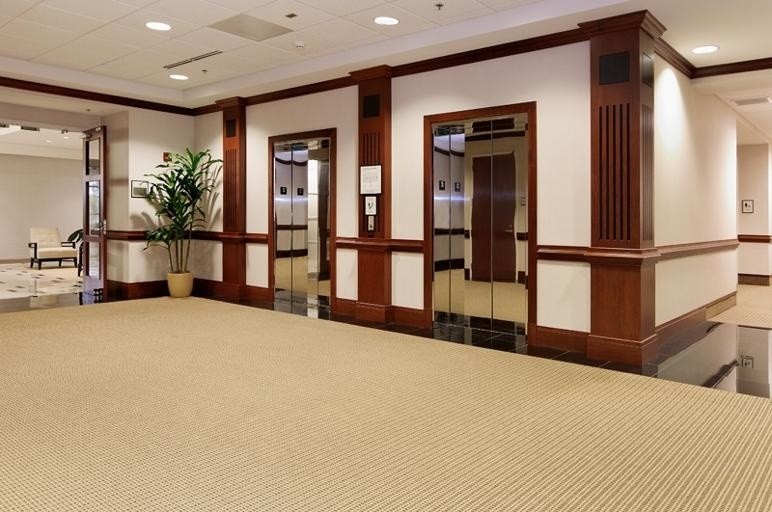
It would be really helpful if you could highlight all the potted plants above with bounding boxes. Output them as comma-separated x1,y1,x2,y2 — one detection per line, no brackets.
139,144,224,298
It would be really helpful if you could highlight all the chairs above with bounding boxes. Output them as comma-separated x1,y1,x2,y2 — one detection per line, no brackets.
28,227,84,276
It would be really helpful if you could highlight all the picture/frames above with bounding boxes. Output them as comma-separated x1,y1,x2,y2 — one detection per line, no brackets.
742,356,753,368
742,200,754,213
